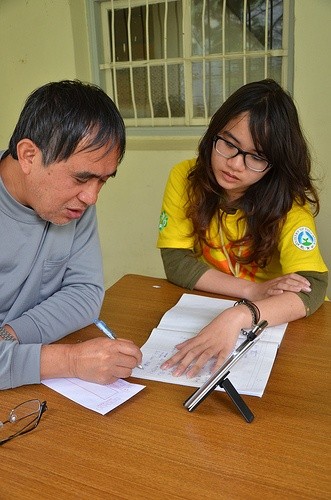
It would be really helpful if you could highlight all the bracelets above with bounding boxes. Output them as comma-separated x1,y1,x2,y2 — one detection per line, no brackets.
0,327,16,342
235,299,260,334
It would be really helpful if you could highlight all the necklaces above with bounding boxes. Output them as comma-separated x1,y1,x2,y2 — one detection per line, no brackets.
217,200,249,279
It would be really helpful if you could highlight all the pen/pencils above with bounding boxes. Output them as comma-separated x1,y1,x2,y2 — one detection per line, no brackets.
93,318,145,372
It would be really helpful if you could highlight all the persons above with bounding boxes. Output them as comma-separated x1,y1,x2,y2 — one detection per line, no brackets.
0,80,142,391
156,80,328,378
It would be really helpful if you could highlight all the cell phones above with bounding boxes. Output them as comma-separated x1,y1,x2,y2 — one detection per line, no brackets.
183,336,260,407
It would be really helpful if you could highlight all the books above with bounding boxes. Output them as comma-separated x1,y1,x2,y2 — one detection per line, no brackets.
132,294,288,397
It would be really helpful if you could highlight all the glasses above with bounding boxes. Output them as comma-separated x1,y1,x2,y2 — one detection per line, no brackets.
212,133,273,173
0,399,48,446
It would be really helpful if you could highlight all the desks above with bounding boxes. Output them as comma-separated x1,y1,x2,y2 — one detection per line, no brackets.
0,274,331,500
